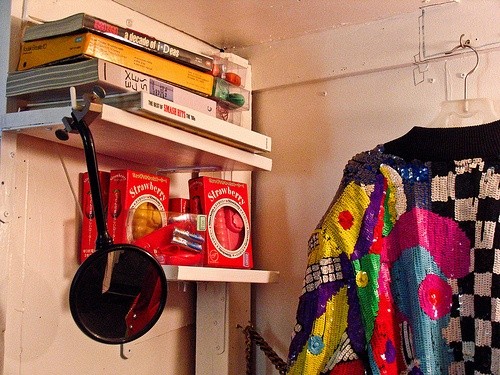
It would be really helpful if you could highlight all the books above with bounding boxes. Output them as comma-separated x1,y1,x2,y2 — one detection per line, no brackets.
5,13,272,154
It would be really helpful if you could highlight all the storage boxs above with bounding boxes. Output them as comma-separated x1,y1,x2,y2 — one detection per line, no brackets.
79,167,253,271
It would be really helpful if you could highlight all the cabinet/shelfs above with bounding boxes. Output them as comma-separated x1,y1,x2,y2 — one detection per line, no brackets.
1,102,279,284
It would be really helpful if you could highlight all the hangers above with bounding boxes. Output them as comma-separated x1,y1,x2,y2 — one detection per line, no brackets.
427,44,498,127
